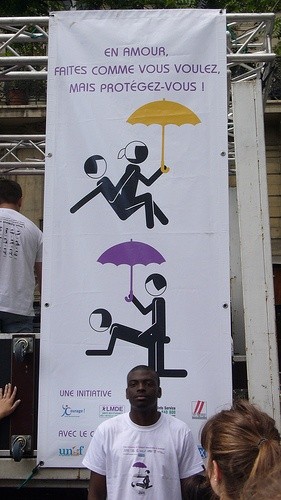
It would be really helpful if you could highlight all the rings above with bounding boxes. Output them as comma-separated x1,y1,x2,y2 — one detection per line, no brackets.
3,396,6,398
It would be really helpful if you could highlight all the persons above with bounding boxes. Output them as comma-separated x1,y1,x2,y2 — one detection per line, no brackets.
201,399,281,500
181,474,211,500
82,366,203,500
0,178,43,450
0,383,21,417
